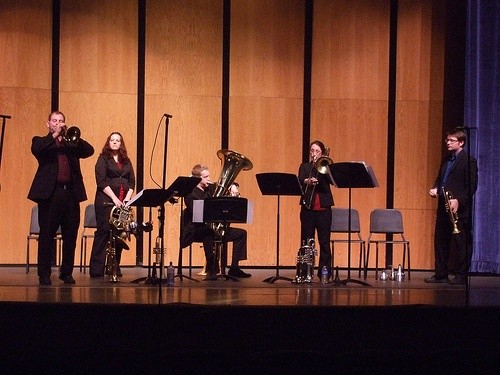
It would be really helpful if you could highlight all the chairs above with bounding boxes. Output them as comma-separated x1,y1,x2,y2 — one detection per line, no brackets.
26,205,62,275
80,204,97,274
366,208,411,281
331,208,366,280
184,208,225,278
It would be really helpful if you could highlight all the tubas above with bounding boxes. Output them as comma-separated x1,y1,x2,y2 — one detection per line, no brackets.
204,147,254,239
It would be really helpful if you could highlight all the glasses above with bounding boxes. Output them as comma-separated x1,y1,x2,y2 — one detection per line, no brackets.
444,138,459,143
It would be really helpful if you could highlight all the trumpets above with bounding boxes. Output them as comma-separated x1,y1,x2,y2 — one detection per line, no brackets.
292,237,315,283
440,185,464,234
63,125,81,141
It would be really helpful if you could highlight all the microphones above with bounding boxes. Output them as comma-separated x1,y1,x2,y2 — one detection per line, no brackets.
164,114,172,118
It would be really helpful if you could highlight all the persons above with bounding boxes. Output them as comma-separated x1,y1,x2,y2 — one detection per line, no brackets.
90,132,135,277
425,130,479,283
297,141,335,283
184,165,252,278
28,111,94,284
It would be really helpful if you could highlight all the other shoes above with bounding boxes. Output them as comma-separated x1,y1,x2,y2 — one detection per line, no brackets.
449,277,468,284
228,269,251,278
39,275,51,285
58,273,75,284
91,272,102,278
207,272,217,280
424,275,450,283
111,271,124,277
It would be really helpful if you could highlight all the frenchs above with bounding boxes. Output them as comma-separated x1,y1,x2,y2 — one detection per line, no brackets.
103,201,136,250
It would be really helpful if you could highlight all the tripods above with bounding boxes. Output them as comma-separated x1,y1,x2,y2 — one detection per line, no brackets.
256,172,304,284
127,175,201,285
328,162,375,287
202,197,248,279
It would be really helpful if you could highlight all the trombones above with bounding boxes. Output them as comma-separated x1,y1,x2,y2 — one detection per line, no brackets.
302,146,334,209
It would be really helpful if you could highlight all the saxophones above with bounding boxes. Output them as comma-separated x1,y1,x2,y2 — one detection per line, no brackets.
103,229,120,282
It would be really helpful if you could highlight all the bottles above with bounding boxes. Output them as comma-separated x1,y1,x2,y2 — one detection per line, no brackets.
321,265,328,285
167,262,174,287
381,264,404,283
